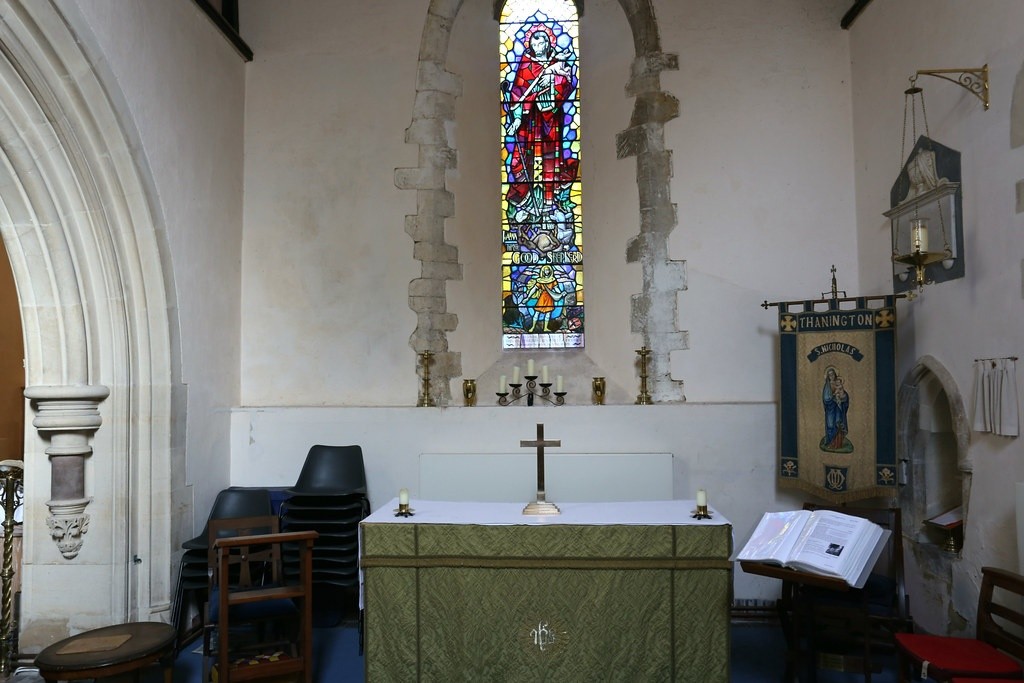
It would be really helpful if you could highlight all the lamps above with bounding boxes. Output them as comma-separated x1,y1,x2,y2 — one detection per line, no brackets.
893,62,991,293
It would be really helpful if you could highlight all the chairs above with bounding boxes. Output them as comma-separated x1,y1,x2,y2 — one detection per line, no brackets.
785,501,914,683
170,445,371,683
895,567,1023,683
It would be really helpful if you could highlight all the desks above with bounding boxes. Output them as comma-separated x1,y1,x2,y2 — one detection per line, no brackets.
35,621,175,683
357,497,734,683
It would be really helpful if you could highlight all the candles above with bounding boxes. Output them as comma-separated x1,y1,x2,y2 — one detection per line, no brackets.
513,366,520,384
399,488,408,504
557,375,563,392
528,358,535,376
500,375,506,393
542,365,549,383
696,490,706,506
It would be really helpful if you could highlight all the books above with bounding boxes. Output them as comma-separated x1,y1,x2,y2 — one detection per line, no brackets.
728,507,892,589
